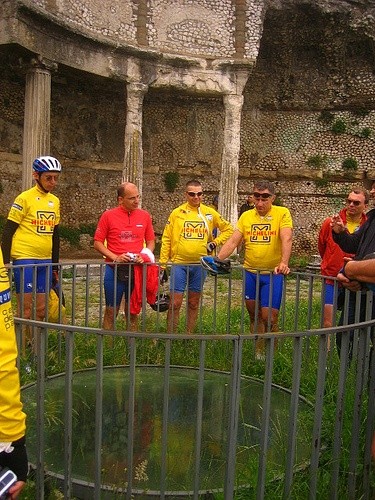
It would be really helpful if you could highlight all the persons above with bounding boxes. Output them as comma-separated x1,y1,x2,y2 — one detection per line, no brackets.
318,188,369,353
239,193,256,218
157,180,235,336
0,184,29,500
0,155,63,359
209,181,295,362
93,182,155,335
329,180,375,368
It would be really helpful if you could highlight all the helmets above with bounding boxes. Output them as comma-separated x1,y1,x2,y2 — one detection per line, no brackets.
32,156,62,173
200,256,230,275
46,288,66,323
150,294,171,311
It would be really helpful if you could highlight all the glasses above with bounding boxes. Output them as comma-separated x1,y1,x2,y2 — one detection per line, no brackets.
346,199,365,205
125,194,142,203
40,175,59,181
187,191,203,197
253,192,272,198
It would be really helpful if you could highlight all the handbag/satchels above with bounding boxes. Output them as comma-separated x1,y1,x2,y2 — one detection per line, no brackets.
115,267,133,283
